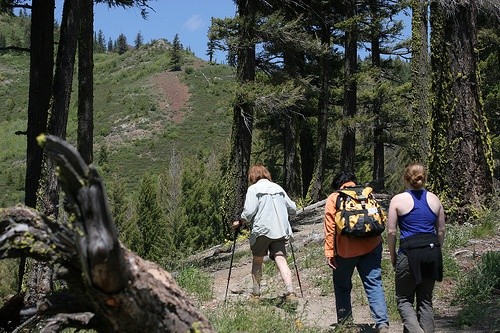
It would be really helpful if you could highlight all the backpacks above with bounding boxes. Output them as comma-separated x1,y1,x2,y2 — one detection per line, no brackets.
335,185,386,238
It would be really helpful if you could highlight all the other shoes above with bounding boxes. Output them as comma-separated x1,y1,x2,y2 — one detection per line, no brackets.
286,292,298,309
248,293,260,307
378,327,388,333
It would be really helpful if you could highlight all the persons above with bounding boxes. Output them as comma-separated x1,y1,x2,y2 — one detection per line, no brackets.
324,171,389,333
387,165,445,333
232,164,296,303
